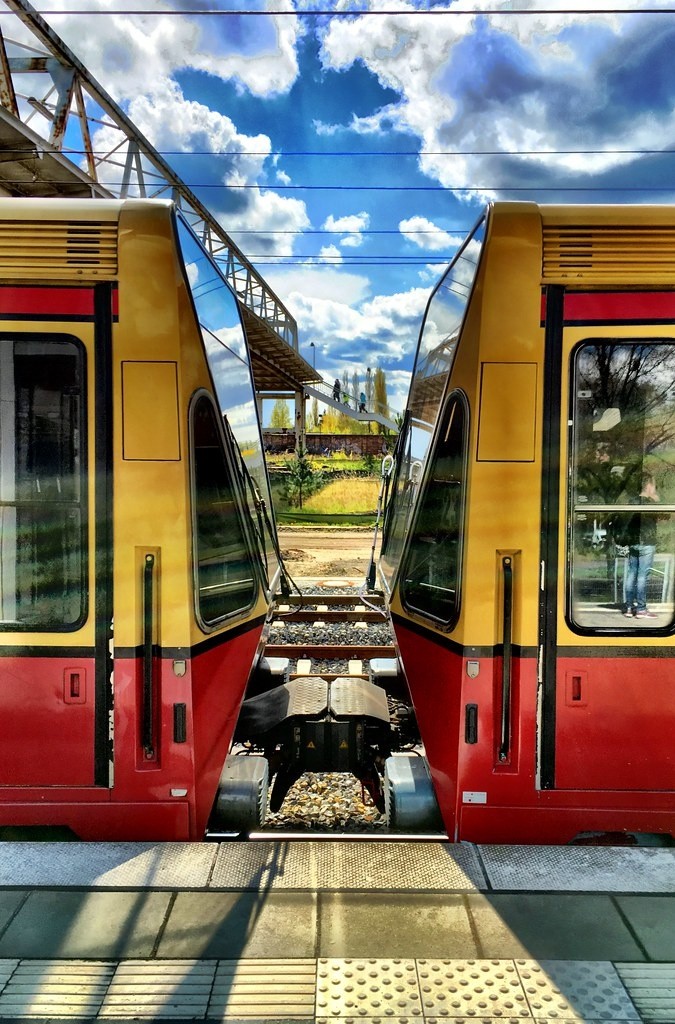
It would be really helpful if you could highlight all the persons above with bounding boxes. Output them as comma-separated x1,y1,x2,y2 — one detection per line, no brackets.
614,472,671,619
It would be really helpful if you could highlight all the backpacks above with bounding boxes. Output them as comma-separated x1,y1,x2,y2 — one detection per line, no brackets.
611,497,641,547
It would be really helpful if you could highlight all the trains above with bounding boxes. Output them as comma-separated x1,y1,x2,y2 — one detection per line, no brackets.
0,195,675,847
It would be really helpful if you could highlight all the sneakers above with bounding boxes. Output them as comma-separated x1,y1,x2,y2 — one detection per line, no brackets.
635,608,658,619
623,606,634,617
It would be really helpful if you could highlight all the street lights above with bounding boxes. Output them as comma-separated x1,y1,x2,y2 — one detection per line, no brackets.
310,342,316,390
